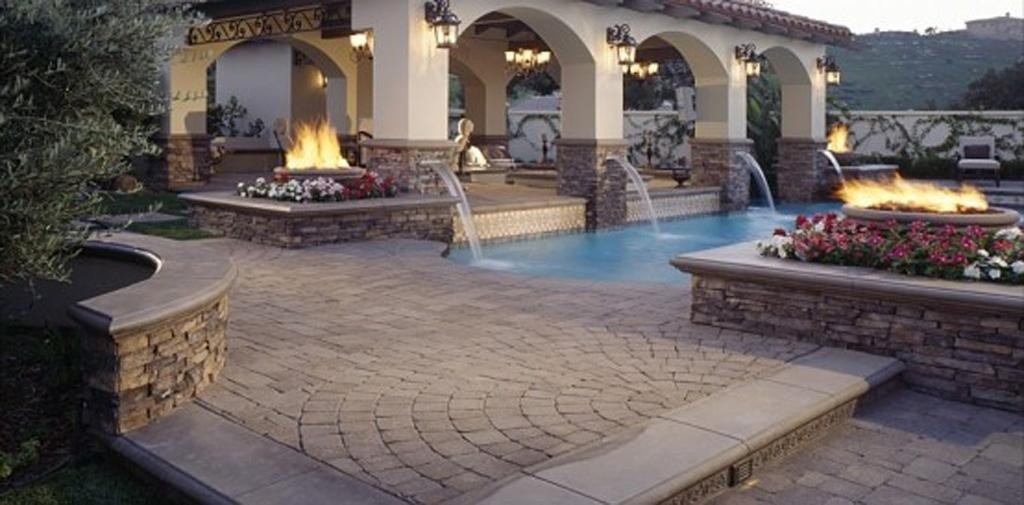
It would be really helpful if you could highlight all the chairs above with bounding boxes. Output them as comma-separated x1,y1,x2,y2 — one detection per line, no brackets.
957,134,1001,186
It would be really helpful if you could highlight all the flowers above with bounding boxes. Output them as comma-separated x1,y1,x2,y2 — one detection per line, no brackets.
760,210,1024,284
237,168,400,203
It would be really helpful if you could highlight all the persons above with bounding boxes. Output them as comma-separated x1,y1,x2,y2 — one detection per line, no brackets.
453,118,488,167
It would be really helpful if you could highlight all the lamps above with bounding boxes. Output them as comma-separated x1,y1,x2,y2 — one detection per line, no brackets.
606,23,640,66
503,22,552,75
735,41,761,76
345,29,373,63
816,52,840,83
423,0,463,50
